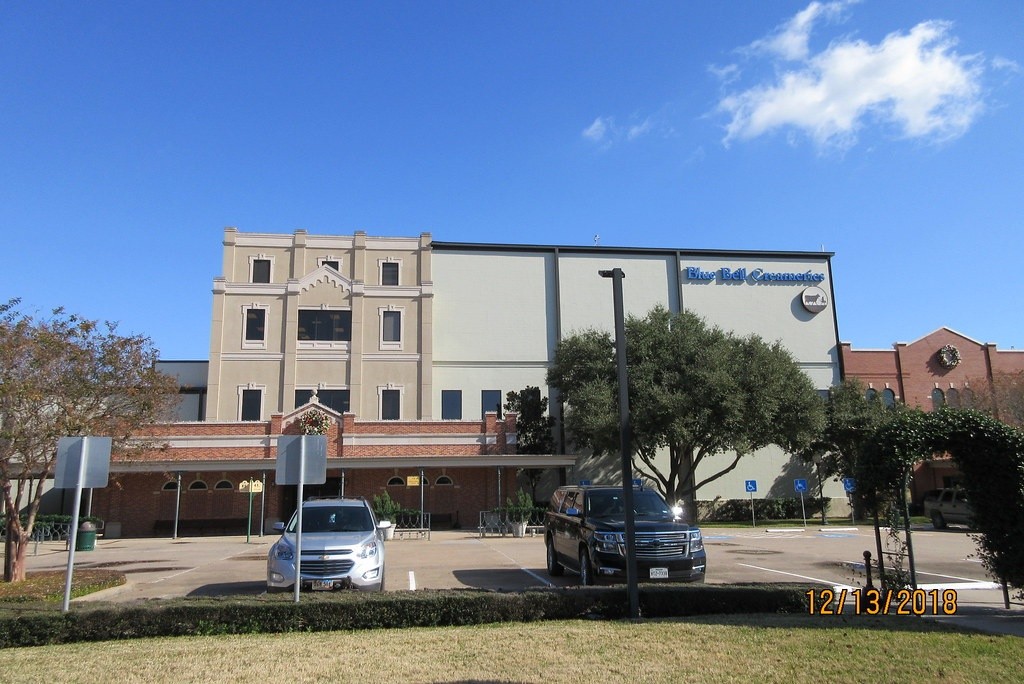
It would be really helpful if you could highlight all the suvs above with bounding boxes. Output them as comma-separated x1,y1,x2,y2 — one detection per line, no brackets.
268,496,392,595
544,484,707,586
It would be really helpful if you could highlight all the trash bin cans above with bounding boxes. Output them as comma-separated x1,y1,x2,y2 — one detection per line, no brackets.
76,521,96,551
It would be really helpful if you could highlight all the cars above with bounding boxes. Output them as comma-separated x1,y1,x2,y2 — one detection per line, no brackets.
923,485,977,531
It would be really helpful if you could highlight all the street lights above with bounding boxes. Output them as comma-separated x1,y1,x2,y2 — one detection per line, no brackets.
598,267,639,618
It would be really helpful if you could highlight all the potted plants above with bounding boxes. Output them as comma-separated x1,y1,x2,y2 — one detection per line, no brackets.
506,486,533,537
372,489,401,541
